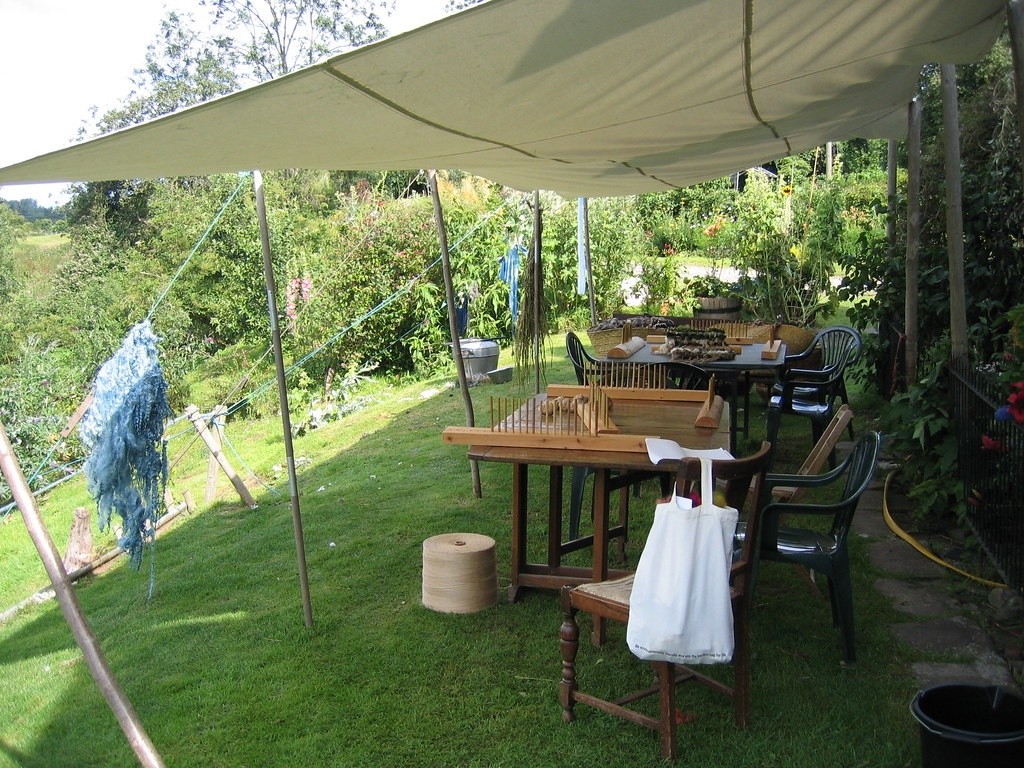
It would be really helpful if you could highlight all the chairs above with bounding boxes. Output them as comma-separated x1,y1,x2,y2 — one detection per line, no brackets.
565,332,750,541
732,428,883,666
763,341,860,472
782,325,861,440
716,404,853,528
559,439,773,760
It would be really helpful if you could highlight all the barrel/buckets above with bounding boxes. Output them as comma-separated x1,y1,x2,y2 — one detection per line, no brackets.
909,681,1024,768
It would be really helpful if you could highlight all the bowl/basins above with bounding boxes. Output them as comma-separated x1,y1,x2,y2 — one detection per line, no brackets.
487,367,513,384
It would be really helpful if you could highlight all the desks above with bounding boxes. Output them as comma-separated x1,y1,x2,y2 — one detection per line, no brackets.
465,393,732,604
597,341,787,470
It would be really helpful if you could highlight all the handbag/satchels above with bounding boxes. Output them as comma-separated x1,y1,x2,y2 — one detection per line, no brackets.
626,457,738,667
